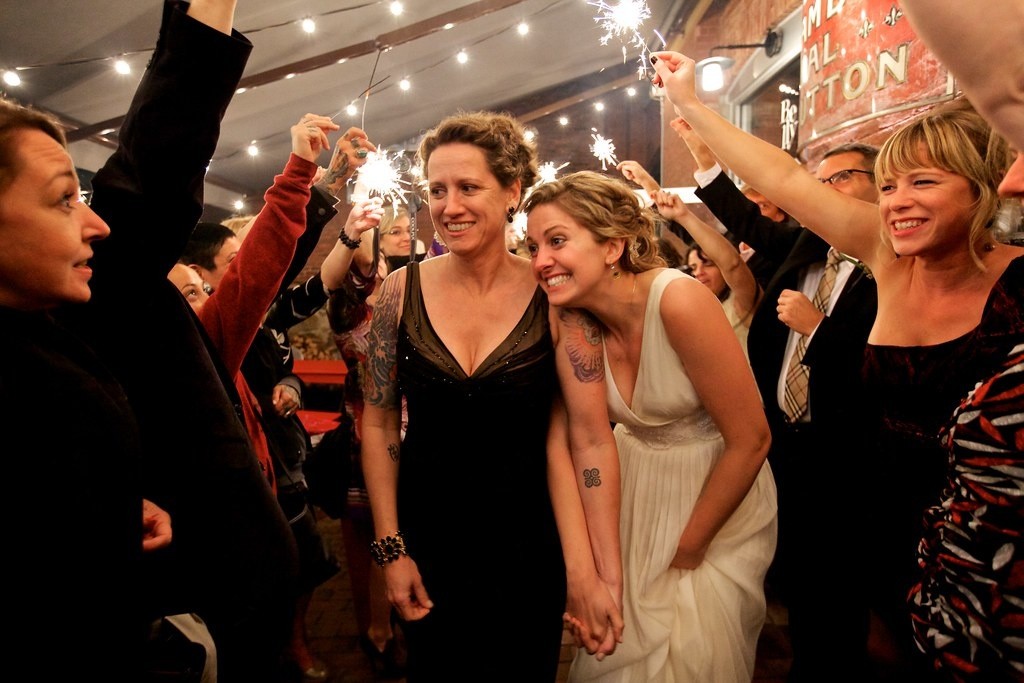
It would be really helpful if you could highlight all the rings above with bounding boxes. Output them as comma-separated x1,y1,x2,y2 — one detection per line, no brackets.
351,137,360,147
357,149,367,158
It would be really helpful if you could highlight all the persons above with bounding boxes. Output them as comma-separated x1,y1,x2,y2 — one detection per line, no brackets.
1,1,1024,683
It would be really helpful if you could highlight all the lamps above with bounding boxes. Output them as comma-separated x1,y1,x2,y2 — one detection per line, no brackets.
696,27,779,91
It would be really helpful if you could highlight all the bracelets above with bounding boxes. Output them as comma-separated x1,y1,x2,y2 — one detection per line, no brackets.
338,227,362,250
370,531,407,568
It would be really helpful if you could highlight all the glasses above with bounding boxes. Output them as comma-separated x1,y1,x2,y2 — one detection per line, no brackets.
818,169,874,185
382,228,411,237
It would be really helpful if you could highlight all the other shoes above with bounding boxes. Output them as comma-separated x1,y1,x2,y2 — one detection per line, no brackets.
288,647,329,678
364,629,396,669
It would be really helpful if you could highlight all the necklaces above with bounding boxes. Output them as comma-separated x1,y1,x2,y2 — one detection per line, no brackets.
626,273,637,314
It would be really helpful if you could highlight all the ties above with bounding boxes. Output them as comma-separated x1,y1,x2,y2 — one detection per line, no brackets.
784,247,843,422
